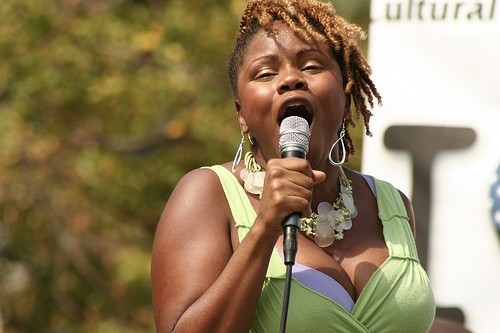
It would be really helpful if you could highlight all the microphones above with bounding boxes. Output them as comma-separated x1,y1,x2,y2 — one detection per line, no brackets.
278,116,312,265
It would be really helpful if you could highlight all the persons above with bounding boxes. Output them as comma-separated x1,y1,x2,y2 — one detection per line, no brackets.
149,0,437,333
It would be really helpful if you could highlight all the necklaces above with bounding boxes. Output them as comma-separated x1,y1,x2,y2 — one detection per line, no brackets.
239,148,358,247
